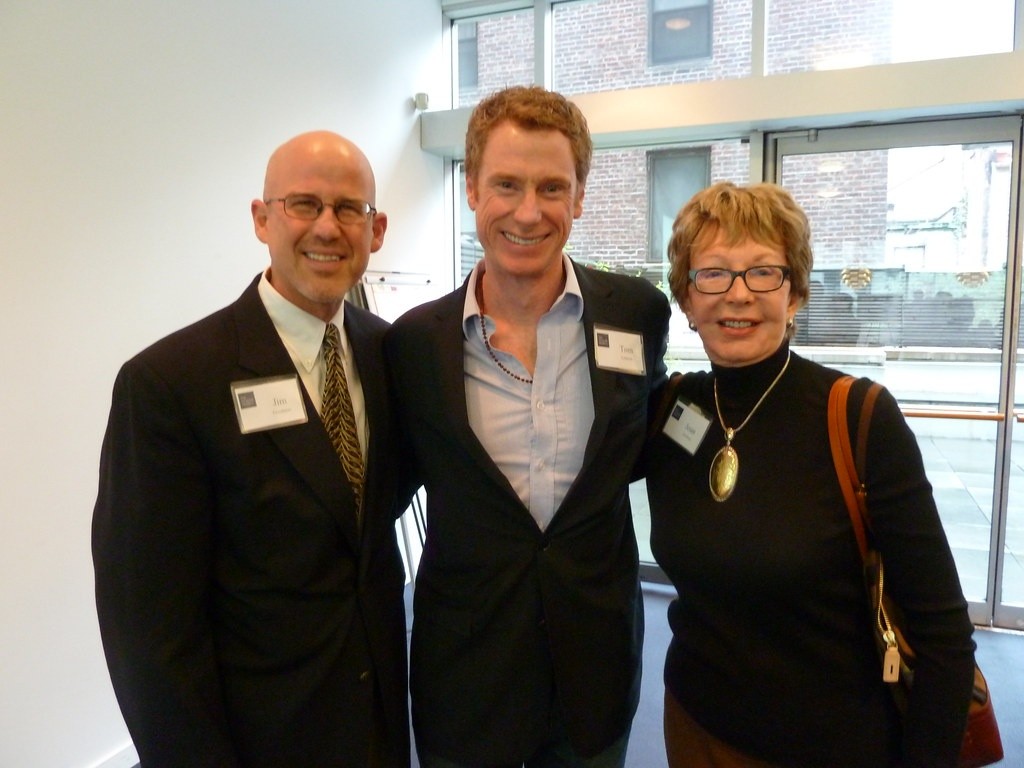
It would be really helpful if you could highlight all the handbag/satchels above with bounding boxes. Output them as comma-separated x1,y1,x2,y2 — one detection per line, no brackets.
827,374,1003,768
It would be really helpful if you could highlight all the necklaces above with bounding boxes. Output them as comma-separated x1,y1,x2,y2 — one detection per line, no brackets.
708,351,790,503
479,294,534,384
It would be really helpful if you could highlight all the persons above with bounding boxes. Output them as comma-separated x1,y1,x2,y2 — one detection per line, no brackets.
91,131,411,768
379,87,670,768
644,182,977,768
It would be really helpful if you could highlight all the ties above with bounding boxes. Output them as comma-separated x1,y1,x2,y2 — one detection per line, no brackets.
318,321,364,499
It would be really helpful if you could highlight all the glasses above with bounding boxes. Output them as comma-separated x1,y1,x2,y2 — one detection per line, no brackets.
262,193,377,226
684,265,792,295
